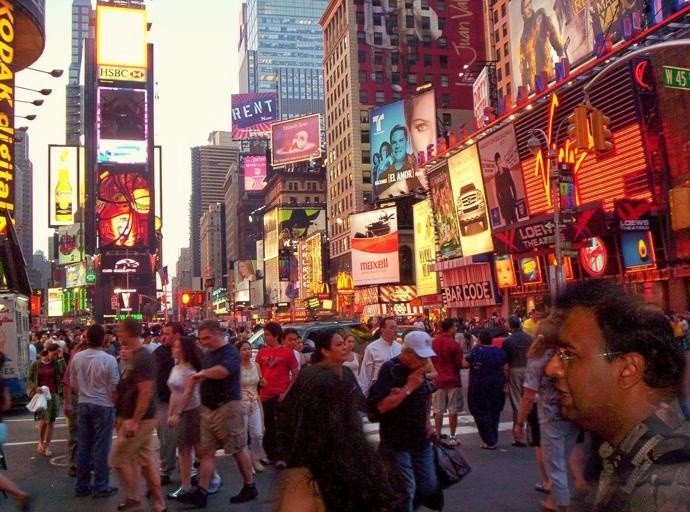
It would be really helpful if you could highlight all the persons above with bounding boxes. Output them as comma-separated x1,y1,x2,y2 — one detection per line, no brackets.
374,124,416,200
0,275,690,511
378,90,436,199
493,151,519,226
376,141,394,179
236,260,257,302
372,153,382,200
517,0,571,93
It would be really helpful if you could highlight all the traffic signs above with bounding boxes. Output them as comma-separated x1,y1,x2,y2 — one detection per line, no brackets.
543,216,581,259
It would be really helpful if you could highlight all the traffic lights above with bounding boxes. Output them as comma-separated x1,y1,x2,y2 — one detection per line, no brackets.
181,292,206,306
567,106,589,152
592,110,615,154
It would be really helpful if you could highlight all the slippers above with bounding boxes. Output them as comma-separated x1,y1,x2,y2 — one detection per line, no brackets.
535,482,573,511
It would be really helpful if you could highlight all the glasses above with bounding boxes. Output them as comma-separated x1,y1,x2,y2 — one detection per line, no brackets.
554,346,624,364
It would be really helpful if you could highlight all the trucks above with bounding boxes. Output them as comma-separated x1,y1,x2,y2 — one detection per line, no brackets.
0,290,32,401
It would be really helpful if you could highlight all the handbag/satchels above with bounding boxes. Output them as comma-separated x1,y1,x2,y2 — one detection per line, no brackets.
432,440,472,489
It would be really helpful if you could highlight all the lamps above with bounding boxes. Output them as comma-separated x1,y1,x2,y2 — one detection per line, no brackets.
458,64,480,83
0,58,64,143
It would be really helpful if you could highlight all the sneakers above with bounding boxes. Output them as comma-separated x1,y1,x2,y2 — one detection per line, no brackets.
204,474,224,493
448,435,527,448
166,485,192,499
156,464,200,487
250,458,287,476
69,463,119,498
36,444,52,458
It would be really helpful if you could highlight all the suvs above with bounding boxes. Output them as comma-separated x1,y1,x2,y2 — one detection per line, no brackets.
456,182,489,235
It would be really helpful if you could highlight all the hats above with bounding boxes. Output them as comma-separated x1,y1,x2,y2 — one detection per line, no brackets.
404,331,438,359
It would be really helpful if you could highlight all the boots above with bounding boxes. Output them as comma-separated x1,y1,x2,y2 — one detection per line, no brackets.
178,490,207,508
231,485,260,504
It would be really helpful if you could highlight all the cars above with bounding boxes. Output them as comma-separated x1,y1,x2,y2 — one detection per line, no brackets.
371,324,438,346
247,322,370,379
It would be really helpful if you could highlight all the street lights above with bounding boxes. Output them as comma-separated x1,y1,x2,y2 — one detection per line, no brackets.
523,125,562,299
